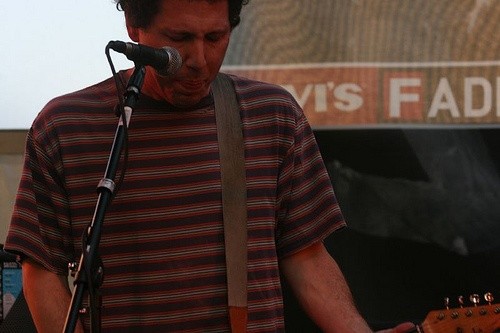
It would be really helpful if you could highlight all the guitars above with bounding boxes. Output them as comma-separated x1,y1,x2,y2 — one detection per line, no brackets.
413,287,500,332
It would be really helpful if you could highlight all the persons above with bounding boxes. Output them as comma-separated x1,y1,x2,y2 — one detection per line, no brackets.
9,0,418,333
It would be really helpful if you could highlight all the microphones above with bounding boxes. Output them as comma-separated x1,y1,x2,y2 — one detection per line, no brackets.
108,40,182,76
